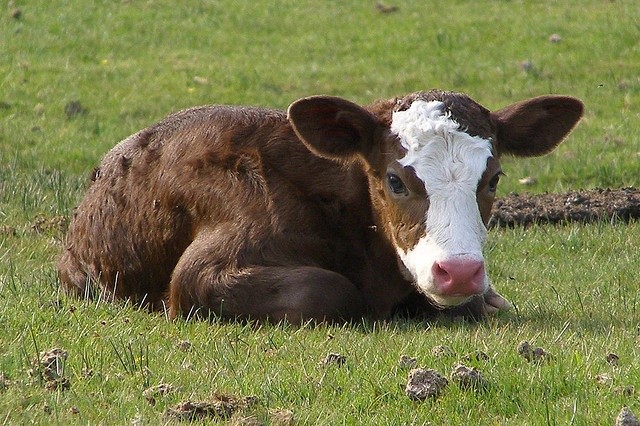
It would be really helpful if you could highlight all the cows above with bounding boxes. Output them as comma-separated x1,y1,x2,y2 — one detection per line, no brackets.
59,87,584,328
